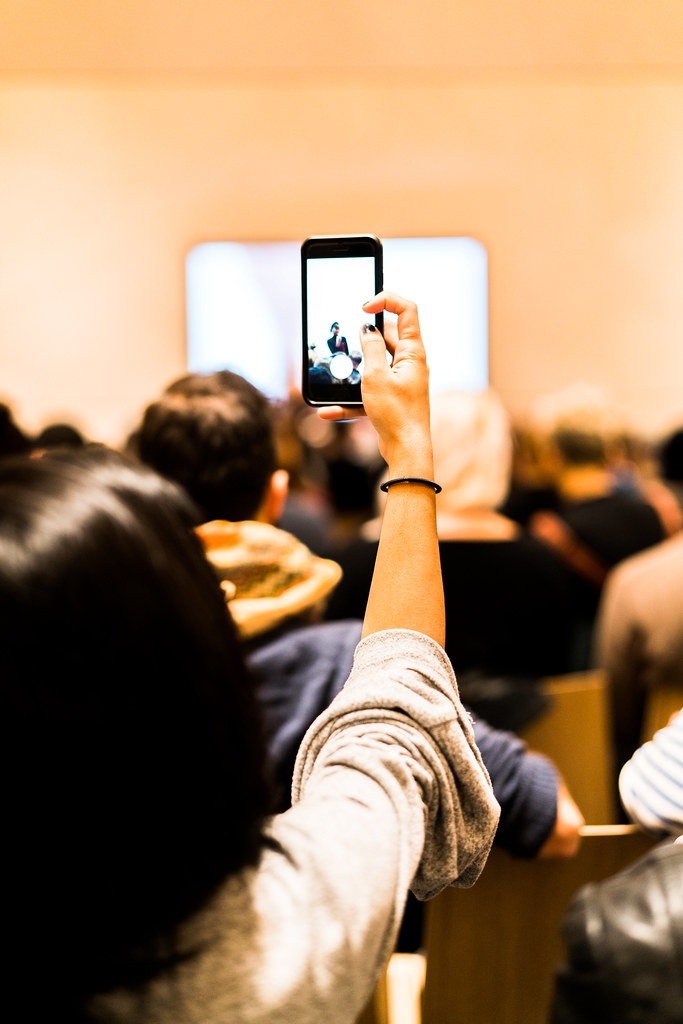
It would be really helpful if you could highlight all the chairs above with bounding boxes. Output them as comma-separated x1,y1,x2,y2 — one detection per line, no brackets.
417,826,666,1023
519,667,683,827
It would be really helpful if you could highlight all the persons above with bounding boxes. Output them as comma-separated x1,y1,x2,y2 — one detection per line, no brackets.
0,285,683,1024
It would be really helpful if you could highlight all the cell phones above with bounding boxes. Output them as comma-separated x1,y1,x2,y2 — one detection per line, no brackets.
301,234,384,407
